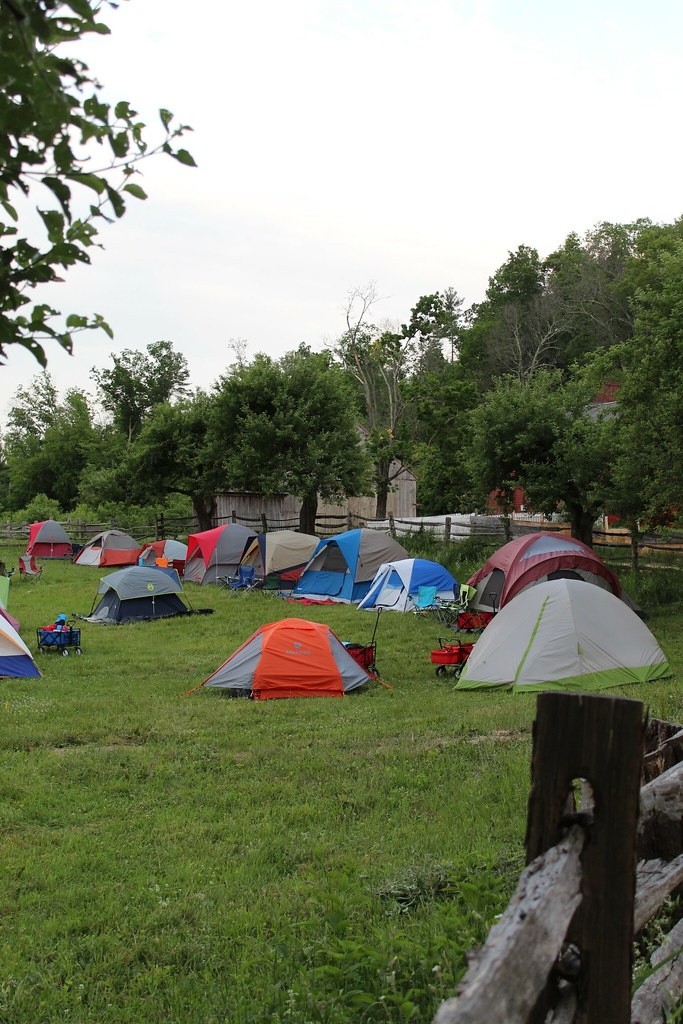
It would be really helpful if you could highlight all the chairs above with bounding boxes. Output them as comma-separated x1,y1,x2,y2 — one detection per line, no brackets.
155,557,168,567
172,558,185,584
63,543,78,563
0,560,14,584
18,555,45,582
439,583,478,627
408,585,442,620
225,564,255,600
261,575,282,598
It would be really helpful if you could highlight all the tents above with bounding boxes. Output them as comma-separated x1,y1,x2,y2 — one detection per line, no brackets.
0,516,675,702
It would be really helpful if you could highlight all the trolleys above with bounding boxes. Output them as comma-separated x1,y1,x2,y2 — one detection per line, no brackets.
341,606,381,682
431,636,476,680
455,592,498,633
36,620,82,657
